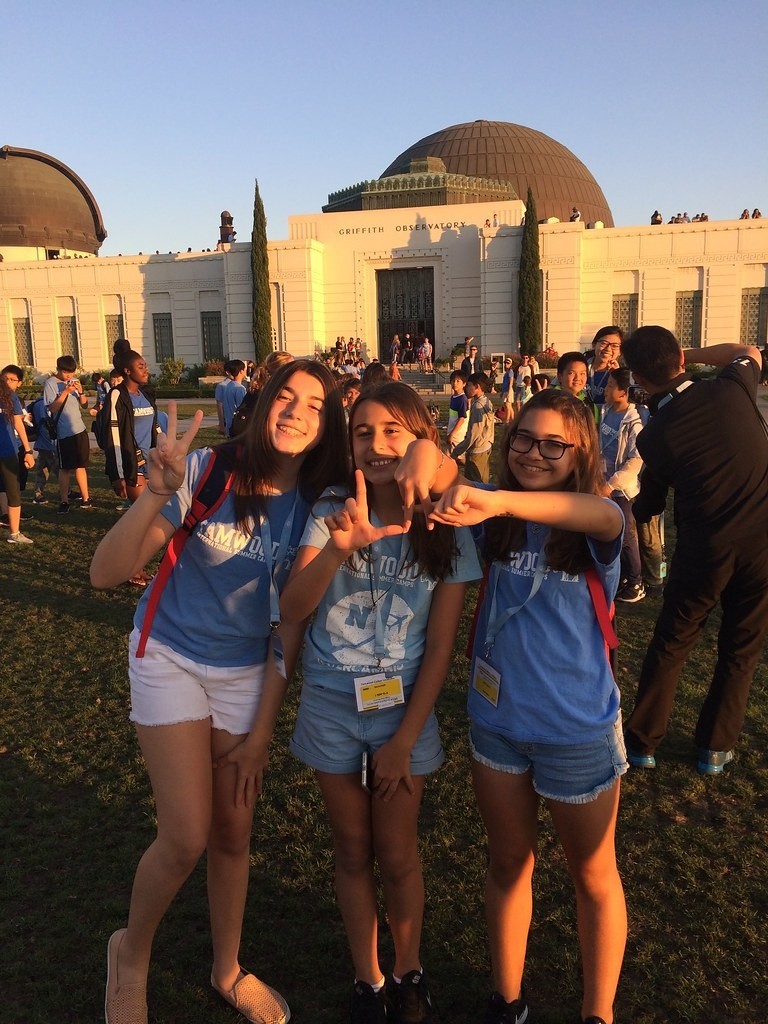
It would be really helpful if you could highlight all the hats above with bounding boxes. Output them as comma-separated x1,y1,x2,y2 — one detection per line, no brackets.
504,358,512,364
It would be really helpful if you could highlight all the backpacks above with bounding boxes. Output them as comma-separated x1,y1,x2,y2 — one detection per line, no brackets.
90,387,120,449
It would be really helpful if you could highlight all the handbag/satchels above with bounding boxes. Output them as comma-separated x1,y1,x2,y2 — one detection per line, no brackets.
417,349,425,360
44,418,58,439
495,405,507,421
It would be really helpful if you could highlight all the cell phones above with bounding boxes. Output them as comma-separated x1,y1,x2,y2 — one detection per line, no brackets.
360,752,374,794
626,385,648,406
72,380,79,386
24,462,29,469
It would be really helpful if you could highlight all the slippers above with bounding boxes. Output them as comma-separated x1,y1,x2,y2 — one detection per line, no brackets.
128,570,155,586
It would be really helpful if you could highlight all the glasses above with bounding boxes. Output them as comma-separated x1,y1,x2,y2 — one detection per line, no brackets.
470,348,477,351
7,377,20,383
597,340,622,350
508,431,573,460
522,357,527,360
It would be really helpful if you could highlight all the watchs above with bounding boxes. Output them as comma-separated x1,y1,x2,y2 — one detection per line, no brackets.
78,388,84,395
24,450,33,454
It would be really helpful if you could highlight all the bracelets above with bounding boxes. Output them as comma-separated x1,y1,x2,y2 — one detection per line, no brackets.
147,483,175,496
436,449,444,471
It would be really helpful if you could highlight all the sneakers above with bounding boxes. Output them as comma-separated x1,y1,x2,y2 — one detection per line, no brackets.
623,756,656,768
577,1012,615,1024
697,747,737,775
0,514,10,526
7,531,33,544
32,490,130,514
615,579,645,602
20,512,34,520
349,976,394,1024
480,987,528,1024
391,964,433,1024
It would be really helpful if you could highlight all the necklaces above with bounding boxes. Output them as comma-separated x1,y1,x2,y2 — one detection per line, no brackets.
531,522,540,535
368,544,413,611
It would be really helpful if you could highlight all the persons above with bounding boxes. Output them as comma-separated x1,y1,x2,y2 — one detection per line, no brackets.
484,219,491,227
622,326,768,774
741,209,749,219
0,333,768,603
395,389,628,1024
752,209,761,219
521,217,525,226
668,212,709,224
492,214,499,227
281,382,485,1024
51,231,237,259
571,207,580,222
89,359,350,1024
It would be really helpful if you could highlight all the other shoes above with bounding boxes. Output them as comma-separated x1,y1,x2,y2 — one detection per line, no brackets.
644,584,663,596
211,963,291,1024
105,929,148,1024
